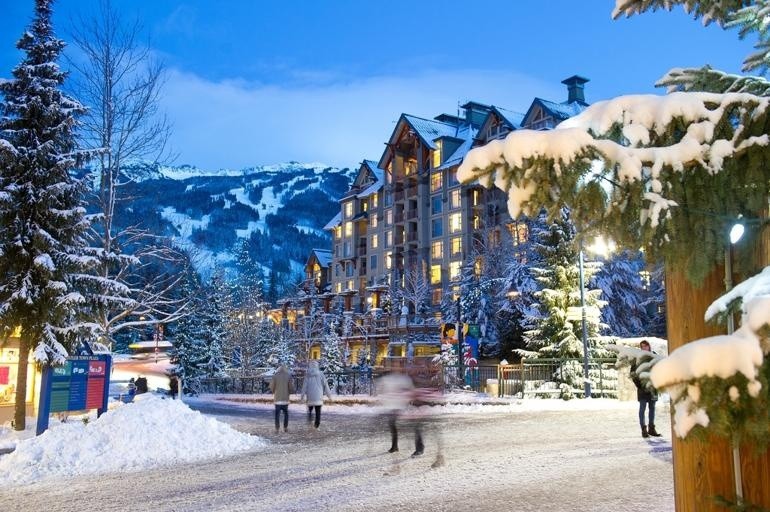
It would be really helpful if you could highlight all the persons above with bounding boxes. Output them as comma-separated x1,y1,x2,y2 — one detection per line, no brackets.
166,374,178,400
135,374,148,395
270,364,293,434
375,360,414,452
301,360,332,430
407,343,446,467
441,323,459,344
631,340,661,437
127,378,136,395
